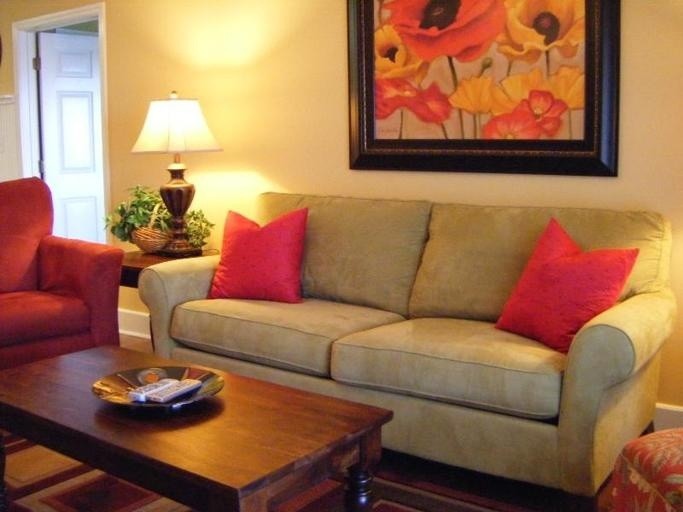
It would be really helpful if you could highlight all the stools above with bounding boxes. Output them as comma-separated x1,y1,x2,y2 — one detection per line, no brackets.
607,425,682,512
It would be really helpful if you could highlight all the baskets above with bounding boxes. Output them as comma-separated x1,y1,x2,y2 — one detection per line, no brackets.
129,202,175,253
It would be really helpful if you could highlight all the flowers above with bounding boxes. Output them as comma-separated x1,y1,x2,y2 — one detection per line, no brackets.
370,2,587,140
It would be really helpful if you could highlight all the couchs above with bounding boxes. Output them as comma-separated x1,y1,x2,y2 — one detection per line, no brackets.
135,192,678,500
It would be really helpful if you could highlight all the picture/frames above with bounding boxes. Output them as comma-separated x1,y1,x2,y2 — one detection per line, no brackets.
347,0,621,177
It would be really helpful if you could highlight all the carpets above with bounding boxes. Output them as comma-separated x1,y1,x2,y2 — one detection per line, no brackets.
0,421,601,512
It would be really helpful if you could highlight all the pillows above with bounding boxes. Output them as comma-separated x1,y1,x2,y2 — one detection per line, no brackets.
207,207,310,308
489,217,649,352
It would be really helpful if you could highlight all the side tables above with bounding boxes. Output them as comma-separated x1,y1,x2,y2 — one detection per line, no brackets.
114,249,204,353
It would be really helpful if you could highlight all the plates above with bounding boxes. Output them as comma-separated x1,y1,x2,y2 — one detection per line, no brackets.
91,366,224,410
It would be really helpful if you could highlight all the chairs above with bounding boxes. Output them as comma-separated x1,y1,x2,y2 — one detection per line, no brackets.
0,175,123,372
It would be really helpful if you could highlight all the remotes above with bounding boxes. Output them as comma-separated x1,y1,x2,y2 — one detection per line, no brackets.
128,379,176,401
149,380,202,402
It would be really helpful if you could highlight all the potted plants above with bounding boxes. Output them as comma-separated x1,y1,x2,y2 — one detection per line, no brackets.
103,181,215,252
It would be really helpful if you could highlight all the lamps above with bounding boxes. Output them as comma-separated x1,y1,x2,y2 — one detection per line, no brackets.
130,86,225,257
